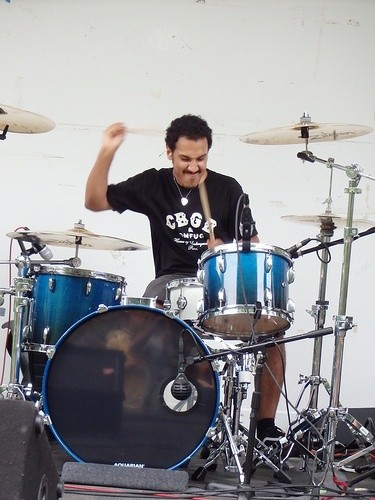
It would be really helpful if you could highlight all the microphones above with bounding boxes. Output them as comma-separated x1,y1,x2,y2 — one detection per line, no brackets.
286,238,311,255
171,329,192,400
24,227,53,260
240,193,255,237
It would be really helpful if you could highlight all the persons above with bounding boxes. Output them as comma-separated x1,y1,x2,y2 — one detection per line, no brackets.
84,115,303,458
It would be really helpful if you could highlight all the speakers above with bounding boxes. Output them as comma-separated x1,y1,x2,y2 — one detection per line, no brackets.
333,408,375,447
0,398,62,500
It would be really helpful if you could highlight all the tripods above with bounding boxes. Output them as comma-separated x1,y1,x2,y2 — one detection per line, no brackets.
190,150,375,485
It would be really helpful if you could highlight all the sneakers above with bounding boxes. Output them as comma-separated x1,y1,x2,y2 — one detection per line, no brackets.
256,426,288,446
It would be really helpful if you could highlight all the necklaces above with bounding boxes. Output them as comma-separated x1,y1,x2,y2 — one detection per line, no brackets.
174,178,193,206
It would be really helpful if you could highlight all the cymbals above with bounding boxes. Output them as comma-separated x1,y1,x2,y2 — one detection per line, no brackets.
281,212,371,225
0,103,55,136
239,119,374,144
6,219,149,255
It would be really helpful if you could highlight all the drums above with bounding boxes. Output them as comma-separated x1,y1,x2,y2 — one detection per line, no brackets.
38,301,224,474
198,243,298,337
18,264,128,354
163,275,208,326
